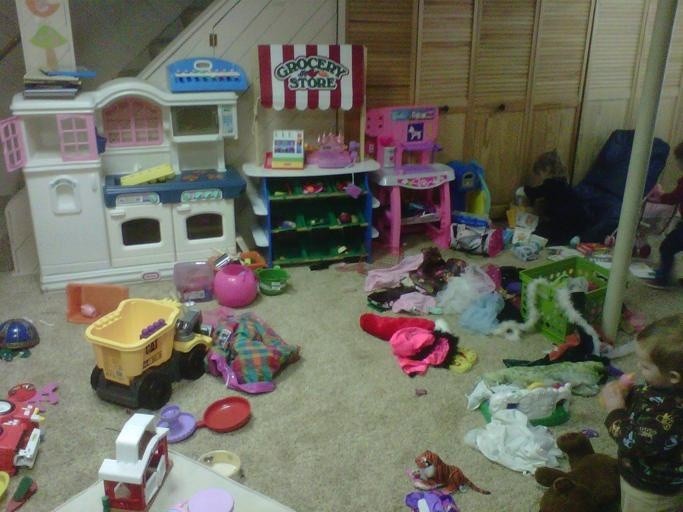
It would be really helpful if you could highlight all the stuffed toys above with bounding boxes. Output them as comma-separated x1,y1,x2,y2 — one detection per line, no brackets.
412,449,492,498
535,431,620,512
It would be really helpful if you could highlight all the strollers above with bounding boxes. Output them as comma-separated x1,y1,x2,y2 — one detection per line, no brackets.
604,183,678,258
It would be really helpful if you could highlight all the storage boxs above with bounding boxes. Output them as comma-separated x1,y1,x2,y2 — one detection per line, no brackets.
519,256,610,346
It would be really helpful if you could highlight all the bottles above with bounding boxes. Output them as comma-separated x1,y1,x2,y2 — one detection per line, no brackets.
596,372,635,409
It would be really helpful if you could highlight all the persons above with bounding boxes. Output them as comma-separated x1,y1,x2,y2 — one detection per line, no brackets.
515,149,584,246
599,313,683,512
642,143,683,289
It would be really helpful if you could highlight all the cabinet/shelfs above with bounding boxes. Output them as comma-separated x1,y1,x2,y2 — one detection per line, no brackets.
346,0,478,173
366,102,455,256
572,0,683,182
468,0,596,219
242,156,380,267
1,57,251,293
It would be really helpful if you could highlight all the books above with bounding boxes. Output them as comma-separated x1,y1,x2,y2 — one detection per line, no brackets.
22,68,82,98
